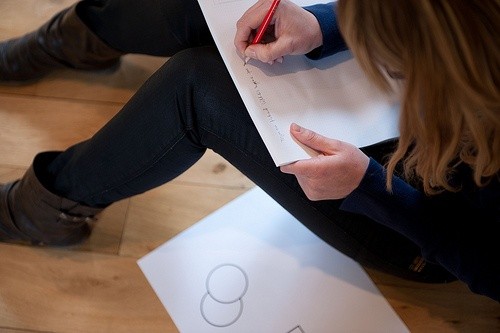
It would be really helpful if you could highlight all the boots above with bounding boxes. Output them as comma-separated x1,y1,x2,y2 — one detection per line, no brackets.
0,151,106,246
0,0,128,85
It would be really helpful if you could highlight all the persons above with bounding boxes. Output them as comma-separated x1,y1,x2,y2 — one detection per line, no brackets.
0,0,500,303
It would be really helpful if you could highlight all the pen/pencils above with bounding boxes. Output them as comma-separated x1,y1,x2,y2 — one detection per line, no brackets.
243,0,281,66
